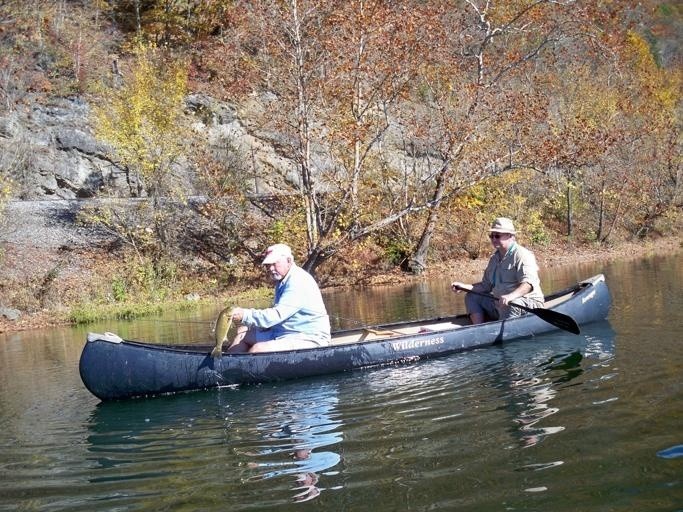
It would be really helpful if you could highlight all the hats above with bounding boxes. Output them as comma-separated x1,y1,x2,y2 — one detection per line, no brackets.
261,244,291,265
485,217,517,235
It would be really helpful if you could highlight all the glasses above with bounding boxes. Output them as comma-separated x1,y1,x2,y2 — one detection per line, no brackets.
488,234,508,238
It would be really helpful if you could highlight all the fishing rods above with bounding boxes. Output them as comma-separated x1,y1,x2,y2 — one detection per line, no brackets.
257,297,410,336
136,318,217,323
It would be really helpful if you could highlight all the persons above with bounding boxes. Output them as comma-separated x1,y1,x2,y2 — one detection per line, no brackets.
451,216,545,325
223,243,332,355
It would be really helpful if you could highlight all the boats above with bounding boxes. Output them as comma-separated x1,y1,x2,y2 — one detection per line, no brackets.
76,271,611,404
80,320,617,472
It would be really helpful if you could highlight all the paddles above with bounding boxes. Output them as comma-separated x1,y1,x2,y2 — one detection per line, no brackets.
452,284,581,335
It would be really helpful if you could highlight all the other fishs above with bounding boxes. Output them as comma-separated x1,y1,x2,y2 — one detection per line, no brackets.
209,306,234,360
